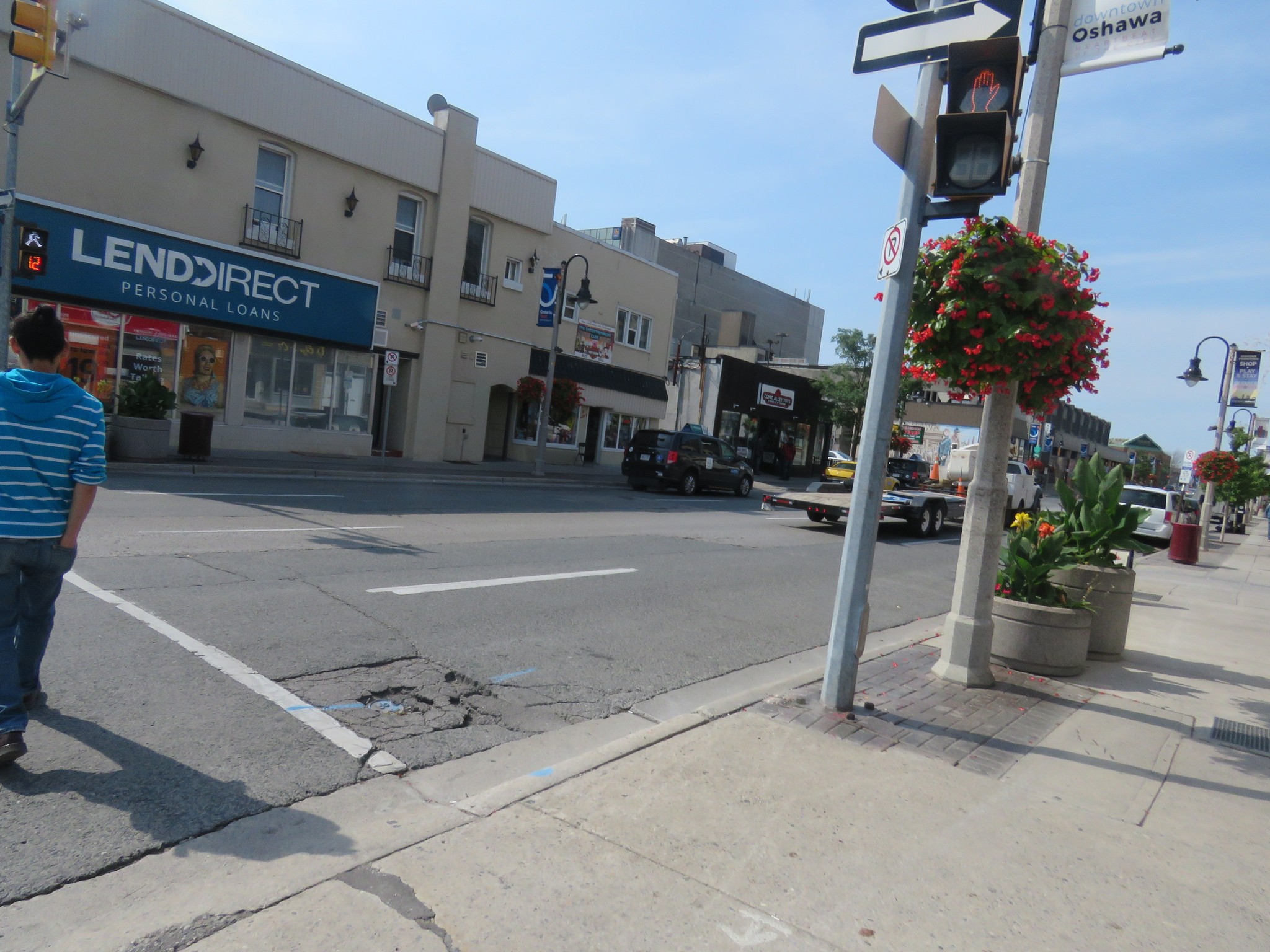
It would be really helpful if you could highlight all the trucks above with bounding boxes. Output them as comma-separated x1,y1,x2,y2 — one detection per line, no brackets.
761,441,1044,538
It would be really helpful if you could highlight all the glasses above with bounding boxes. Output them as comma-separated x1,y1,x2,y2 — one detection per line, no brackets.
196,356,215,364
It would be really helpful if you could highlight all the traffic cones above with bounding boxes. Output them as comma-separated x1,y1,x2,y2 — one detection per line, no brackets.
957,477,966,498
929,456,940,481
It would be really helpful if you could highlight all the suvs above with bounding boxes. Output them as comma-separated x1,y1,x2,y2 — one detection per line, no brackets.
621,429,755,498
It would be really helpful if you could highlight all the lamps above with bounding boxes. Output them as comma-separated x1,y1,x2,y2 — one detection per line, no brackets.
187,131,205,169
344,185,360,217
529,249,540,273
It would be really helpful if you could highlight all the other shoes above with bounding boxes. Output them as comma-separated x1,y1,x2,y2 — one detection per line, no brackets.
0,731,27,763
22,689,40,710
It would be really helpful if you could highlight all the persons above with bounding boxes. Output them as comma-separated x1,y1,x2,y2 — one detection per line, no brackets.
0,306,106,762
1265,503,1270,541
933,427,960,467
779,437,796,480
180,344,224,409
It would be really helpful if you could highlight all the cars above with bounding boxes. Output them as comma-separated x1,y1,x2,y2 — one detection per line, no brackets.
1117,485,1201,542
821,450,901,491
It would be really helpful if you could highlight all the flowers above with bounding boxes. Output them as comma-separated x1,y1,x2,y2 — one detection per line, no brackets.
873,211,1114,423
1025,458,1055,474
1193,449,1240,485
891,436,913,454
994,510,1103,617
515,376,586,411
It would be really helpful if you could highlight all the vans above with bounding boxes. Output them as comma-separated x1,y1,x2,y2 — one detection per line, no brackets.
887,457,935,486
1192,486,1231,523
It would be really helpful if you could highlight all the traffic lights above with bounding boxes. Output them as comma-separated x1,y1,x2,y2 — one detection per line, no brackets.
19,226,51,253
945,36,1024,117
9,0,59,69
932,111,1015,199
18,250,49,278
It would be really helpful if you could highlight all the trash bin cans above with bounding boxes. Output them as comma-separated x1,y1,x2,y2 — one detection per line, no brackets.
178,409,213,460
1167,522,1203,565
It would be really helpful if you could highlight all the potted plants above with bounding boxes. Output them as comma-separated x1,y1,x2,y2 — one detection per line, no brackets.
1045,452,1163,654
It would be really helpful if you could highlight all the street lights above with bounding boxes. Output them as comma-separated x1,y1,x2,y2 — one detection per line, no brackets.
1226,408,1257,533
1045,432,1064,494
1176,336,1237,552
531,253,599,478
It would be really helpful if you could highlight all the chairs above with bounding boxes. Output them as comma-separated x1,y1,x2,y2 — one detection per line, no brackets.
574,442,594,469
